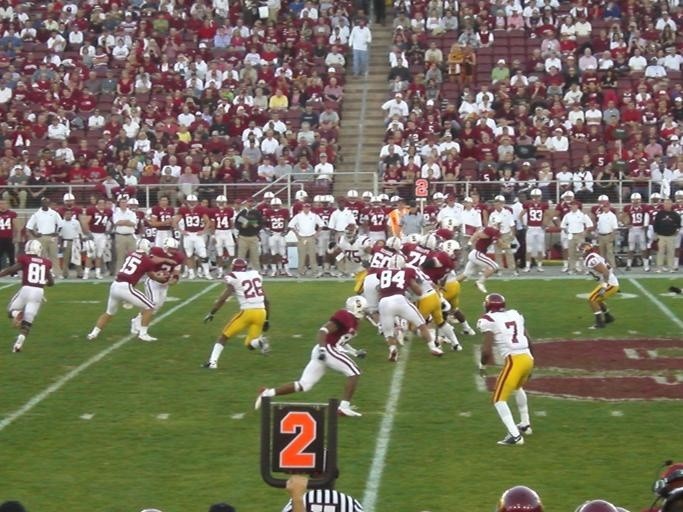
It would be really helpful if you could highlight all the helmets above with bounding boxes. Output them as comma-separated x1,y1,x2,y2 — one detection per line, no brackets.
420,234,435,250
187,195,198,208
264,192,274,202
564,190,574,203
391,197,400,205
578,501,619,512
370,196,382,204
483,293,505,313
386,237,399,250
651,193,663,204
314,195,324,204
270,198,282,211
408,234,421,243
216,196,227,208
347,190,358,204
380,194,389,203
294,191,308,203
495,196,505,203
597,195,608,205
577,243,593,255
136,239,151,255
363,191,372,202
345,296,367,318
491,220,501,231
530,189,543,201
442,240,461,259
163,239,177,254
64,193,76,203
498,486,543,512
231,259,248,271
631,192,641,205
344,224,359,239
675,191,683,203
24,241,42,257
390,255,402,268
323,195,333,204
433,192,443,204
127,198,139,207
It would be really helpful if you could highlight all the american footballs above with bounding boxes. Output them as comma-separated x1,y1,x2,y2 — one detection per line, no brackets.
157,271,170,282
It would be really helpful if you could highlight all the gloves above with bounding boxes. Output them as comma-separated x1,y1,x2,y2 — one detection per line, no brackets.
465,244,472,252
203,312,214,323
479,362,487,378
509,243,517,249
598,284,608,297
318,349,326,360
356,349,366,357
263,321,269,331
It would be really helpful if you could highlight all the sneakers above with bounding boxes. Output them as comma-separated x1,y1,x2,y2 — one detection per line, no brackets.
337,406,361,418
475,281,487,293
496,434,524,446
604,317,615,324
589,323,606,329
12,312,23,328
13,343,22,352
87,335,96,341
201,362,219,369
259,338,271,356
430,349,445,356
453,345,463,351
395,318,424,345
377,325,384,335
462,329,474,335
254,388,269,410
130,321,139,335
517,426,531,434
138,334,157,343
389,347,397,361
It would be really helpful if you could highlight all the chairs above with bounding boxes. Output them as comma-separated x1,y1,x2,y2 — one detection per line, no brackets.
68,143,78,153
64,51,78,58
668,71,682,78
511,53,526,64
589,142,601,153
616,78,631,87
494,46,509,53
87,129,102,138
32,144,46,155
34,51,45,60
477,47,493,55
592,27,607,37
572,148,587,160
66,43,82,51
553,150,570,161
618,86,631,97
591,19,604,27
71,179,85,204
572,156,583,172
443,29,460,38
286,109,304,118
475,80,490,91
134,94,149,106
460,168,478,181
443,46,452,53
461,160,479,170
99,94,114,104
426,37,441,47
509,35,525,47
442,37,456,46
442,89,459,98
577,36,592,45
494,36,510,45
509,29,526,37
526,36,540,45
442,81,459,90
493,30,507,37
71,130,85,140
285,117,301,133
571,142,586,151
493,52,510,64
32,139,49,147
476,62,493,72
476,53,493,63
97,102,112,112
527,43,539,53
554,158,571,173
476,71,491,81
671,79,683,89
23,42,35,51
35,44,45,51
87,144,99,153
85,135,99,146
409,65,426,73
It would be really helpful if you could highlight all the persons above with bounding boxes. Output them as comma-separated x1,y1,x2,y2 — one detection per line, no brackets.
343,190,363,234
476,293,535,445
578,244,619,329
262,198,292,277
0,240,54,353
150,196,175,247
432,192,445,229
386,196,404,214
377,193,391,240
396,200,429,235
174,194,214,280
145,207,158,246
423,193,443,230
131,237,187,336
552,190,582,272
82,199,114,279
457,197,482,270
114,198,139,277
365,197,386,241
327,196,357,278
592,194,616,254
310,195,323,267
20,222,26,242
458,212,519,294
642,465,683,511
211,195,236,279
313,194,336,272
287,476,309,512
292,189,318,269
596,203,617,272
256,191,285,275
377,0,462,204
359,192,373,234
623,192,650,272
488,200,519,276
386,200,410,237
512,192,535,268
490,0,683,201
560,201,594,276
200,199,218,270
520,188,553,274
355,228,474,361
463,192,489,227
0,0,387,206
287,202,323,278
483,195,514,267
457,0,499,192
437,193,462,230
237,198,261,272
231,200,242,241
384,192,463,268
254,295,368,418
86,195,98,215
201,258,271,369
86,238,158,341
648,190,683,274
56,193,85,272
25,198,63,279
0,201,19,278
50,202,63,220
283,465,365,512
